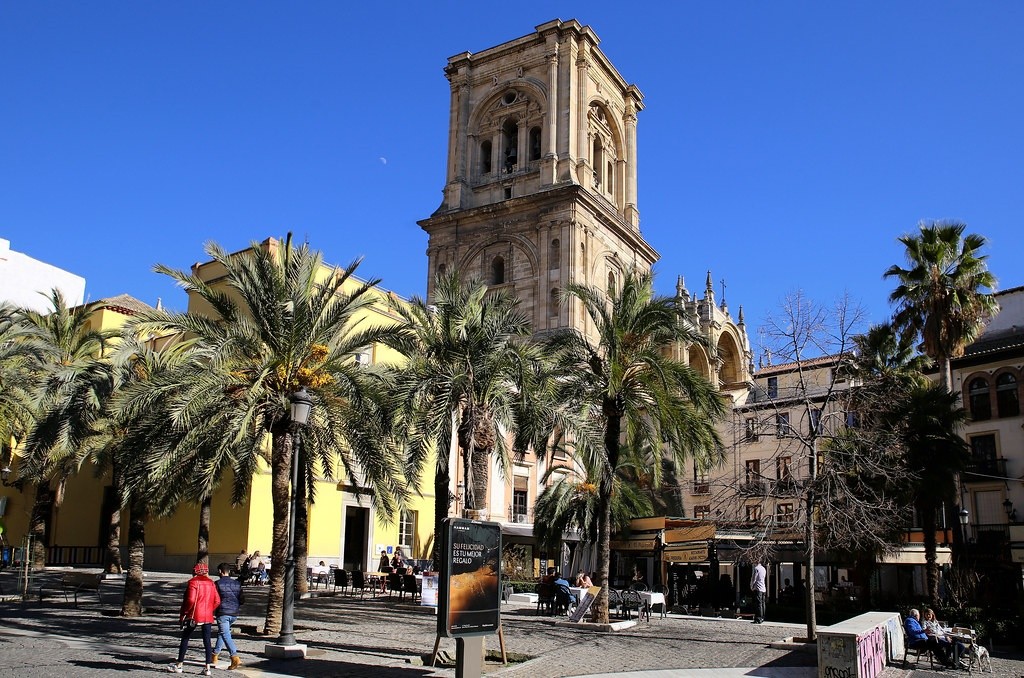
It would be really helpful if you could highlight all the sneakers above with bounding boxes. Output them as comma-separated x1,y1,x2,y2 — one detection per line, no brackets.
166,663,183,673
202,668,212,676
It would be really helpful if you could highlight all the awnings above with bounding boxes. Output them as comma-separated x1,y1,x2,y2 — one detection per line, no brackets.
876,545,952,563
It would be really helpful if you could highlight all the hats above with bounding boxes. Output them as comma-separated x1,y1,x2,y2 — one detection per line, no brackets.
192,563,208,576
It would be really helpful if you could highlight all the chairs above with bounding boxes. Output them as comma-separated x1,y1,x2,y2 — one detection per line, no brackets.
232,558,670,621
899,625,946,669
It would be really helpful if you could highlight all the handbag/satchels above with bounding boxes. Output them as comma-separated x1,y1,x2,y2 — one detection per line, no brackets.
181,618,196,630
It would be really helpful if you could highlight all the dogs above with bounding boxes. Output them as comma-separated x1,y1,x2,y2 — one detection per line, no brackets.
968,643,992,676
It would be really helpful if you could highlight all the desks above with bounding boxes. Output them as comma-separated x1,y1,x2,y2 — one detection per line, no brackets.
945,632,976,669
624,591,665,620
416,575,422,588
569,587,589,603
362,572,389,597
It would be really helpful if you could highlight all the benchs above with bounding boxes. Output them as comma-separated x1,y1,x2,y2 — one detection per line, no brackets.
40,568,106,608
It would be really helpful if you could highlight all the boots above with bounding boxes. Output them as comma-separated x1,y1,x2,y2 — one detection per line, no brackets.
228,655,242,670
210,652,220,665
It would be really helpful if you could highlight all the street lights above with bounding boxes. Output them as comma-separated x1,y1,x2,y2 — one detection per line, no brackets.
262,385,320,661
958,508,969,543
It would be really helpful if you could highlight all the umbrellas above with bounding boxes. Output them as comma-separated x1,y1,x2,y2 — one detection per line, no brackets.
561,541,598,587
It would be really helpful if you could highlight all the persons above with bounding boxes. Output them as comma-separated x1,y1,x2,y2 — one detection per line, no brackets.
904,608,966,670
377,546,415,588
236,549,272,586
784,578,793,594
749,556,766,624
166,563,220,675
535,567,594,617
212,562,245,670
